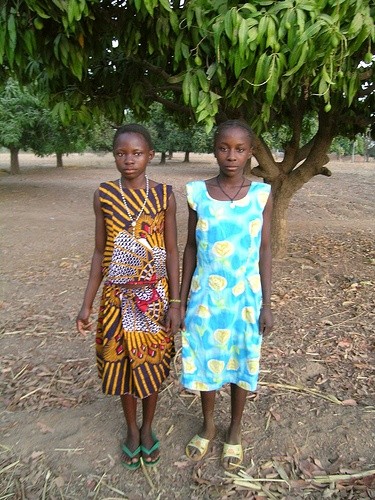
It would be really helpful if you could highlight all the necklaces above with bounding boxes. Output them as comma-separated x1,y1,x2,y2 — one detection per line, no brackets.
217,174,245,204
118,173,149,241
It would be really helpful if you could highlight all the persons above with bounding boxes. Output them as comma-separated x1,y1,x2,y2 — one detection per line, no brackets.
179,119,274,470
76,123,181,470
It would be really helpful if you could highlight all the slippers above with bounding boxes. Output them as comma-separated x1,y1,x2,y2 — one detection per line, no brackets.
186,433,209,461
121,442,141,471
221,428,243,469
141,431,161,467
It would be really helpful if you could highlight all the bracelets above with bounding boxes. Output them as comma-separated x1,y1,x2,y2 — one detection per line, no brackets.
262,304,271,307
170,300,181,303
